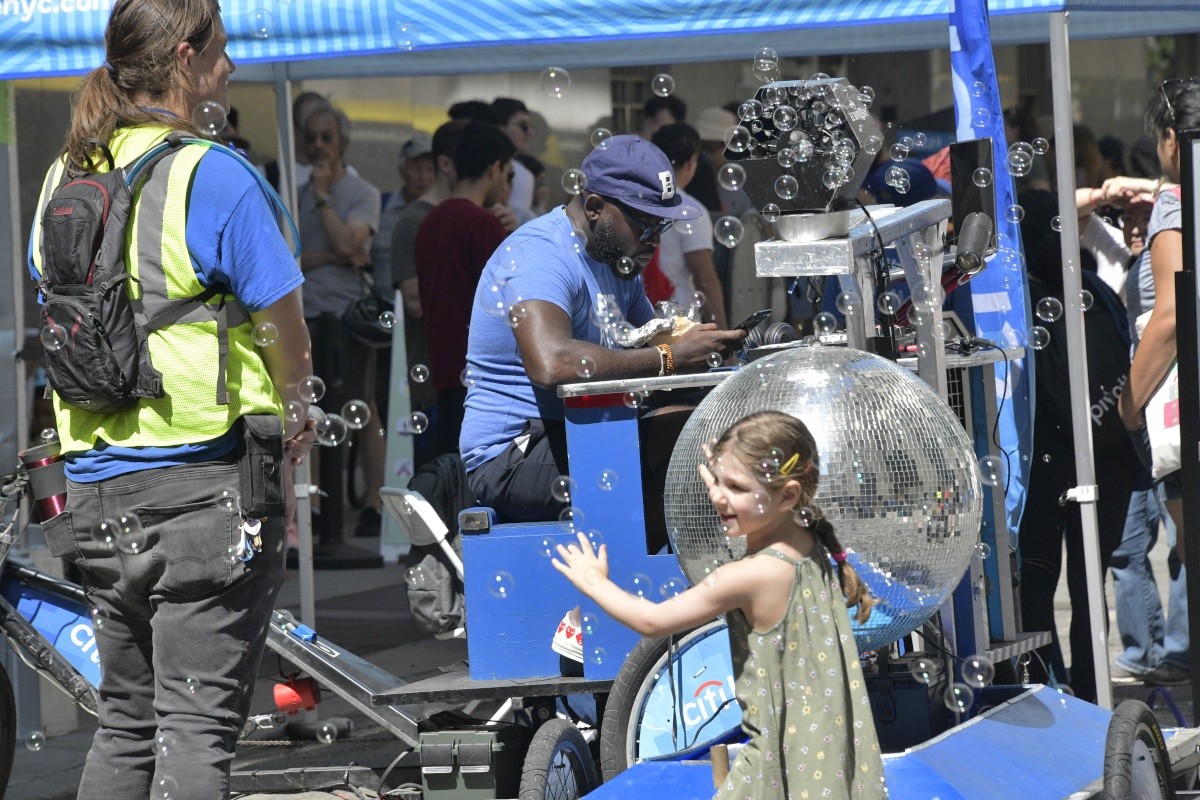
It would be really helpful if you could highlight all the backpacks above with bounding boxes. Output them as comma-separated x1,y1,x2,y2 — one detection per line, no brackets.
32,134,228,413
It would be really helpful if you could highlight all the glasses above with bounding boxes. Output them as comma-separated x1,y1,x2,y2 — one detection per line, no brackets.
606,196,673,241
1158,75,1198,120
511,121,528,133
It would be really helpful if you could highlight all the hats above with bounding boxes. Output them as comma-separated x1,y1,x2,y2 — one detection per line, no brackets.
581,135,703,220
400,140,433,160
863,159,952,206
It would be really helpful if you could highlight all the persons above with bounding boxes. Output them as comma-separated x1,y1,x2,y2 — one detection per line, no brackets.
24,0,316,798
551,408,889,800
209,59,1200,696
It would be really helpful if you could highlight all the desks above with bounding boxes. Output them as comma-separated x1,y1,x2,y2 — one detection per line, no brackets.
557,347,1025,682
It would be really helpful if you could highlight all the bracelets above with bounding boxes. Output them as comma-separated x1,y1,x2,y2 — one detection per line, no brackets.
659,343,675,376
312,198,335,211
653,346,666,376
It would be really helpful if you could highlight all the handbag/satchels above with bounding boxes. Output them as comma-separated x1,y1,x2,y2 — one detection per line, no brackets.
1135,309,1181,479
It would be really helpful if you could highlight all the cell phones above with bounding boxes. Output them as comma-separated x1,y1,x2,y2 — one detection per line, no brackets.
731,308,773,341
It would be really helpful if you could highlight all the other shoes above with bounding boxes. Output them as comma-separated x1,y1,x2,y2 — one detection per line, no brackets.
356,510,382,536
1111,660,1190,685
